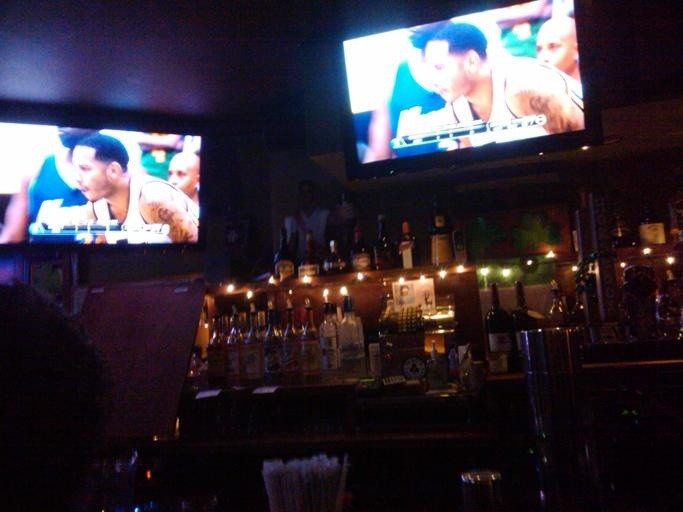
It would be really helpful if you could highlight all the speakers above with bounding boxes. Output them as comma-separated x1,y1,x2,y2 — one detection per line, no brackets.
212,162,272,274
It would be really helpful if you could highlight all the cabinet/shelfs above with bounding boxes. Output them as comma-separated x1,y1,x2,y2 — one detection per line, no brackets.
206,174,683,391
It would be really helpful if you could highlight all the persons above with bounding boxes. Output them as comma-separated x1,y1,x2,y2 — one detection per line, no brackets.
359,18,587,165
0,125,199,244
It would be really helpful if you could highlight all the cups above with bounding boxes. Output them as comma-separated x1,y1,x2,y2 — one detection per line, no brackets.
460,470,506,511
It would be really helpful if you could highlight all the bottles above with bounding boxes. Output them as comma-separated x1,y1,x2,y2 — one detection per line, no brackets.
425,339,458,390
378,300,397,325
487,281,588,374
614,264,683,345
416,303,423,319
263,181,457,277
191,295,368,379
379,280,392,312
607,194,683,250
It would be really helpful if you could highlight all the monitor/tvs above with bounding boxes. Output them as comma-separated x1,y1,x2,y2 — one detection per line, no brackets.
0,111,210,258
333,0,603,182
